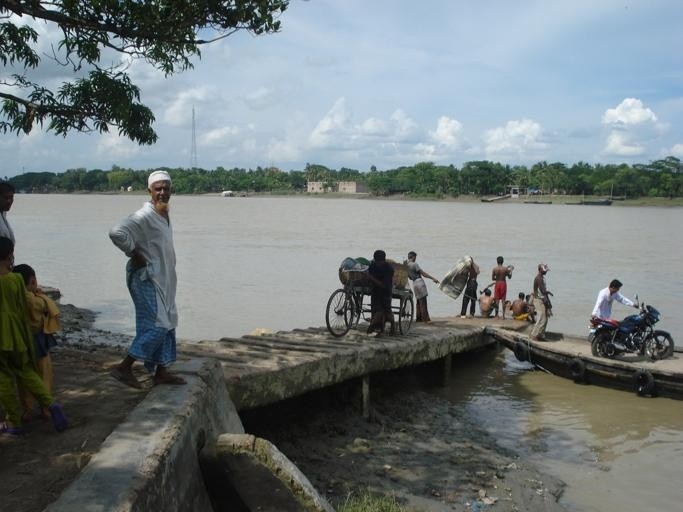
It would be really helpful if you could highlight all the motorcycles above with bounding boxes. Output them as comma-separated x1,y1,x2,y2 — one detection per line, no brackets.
587,293,674,360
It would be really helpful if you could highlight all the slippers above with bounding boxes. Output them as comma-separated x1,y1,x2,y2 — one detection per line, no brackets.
153,376,188,385
110,368,142,389
1,405,67,434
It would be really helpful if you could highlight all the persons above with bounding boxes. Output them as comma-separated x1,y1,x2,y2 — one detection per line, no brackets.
0,234,68,435
529,262,553,342
362,250,394,338
0,181,15,265
456,255,477,319
107,170,188,389
491,256,511,320
478,290,494,317
403,251,440,322
591,278,639,351
10,263,60,420
509,293,535,323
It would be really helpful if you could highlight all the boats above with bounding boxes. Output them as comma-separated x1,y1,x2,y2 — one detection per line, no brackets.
564,203,583,205
583,199,612,206
524,201,537,204
536,201,552,204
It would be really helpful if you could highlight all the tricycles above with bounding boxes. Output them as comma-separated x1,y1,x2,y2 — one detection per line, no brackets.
325,264,414,337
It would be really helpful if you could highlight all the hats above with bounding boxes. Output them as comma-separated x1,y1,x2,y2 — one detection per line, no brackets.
147,171,171,187
538,264,550,272
